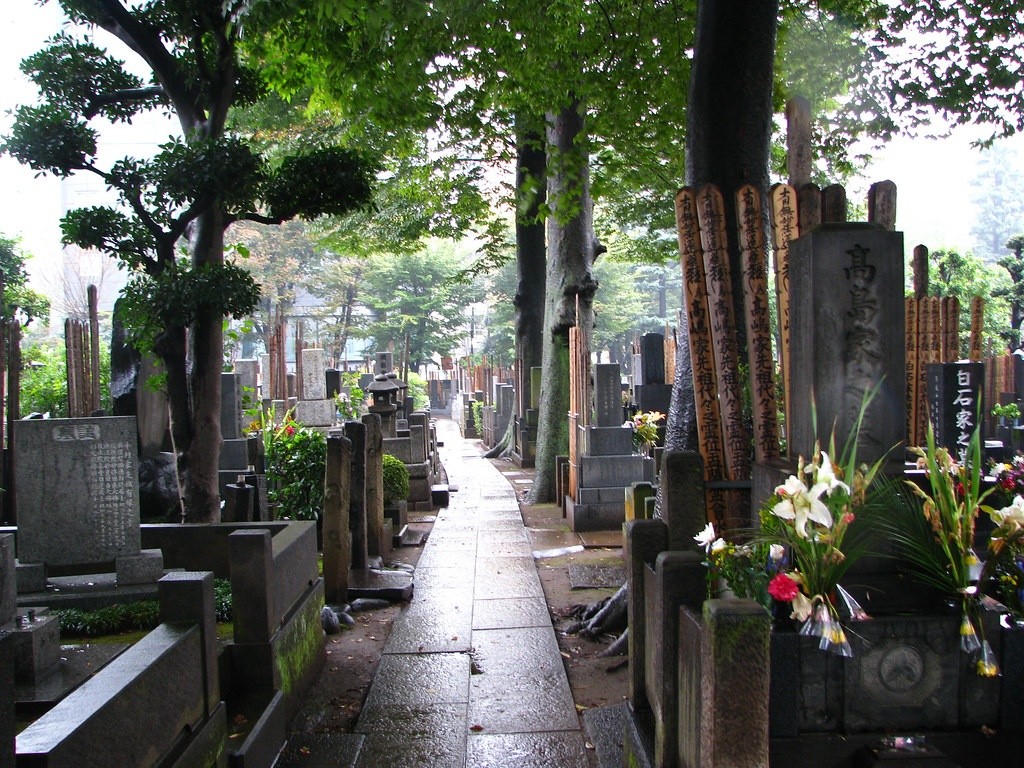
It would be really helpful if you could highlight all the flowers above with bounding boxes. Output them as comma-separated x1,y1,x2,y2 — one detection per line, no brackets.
241,401,302,459
620,410,666,459
691,371,904,657
904,384,1024,678
